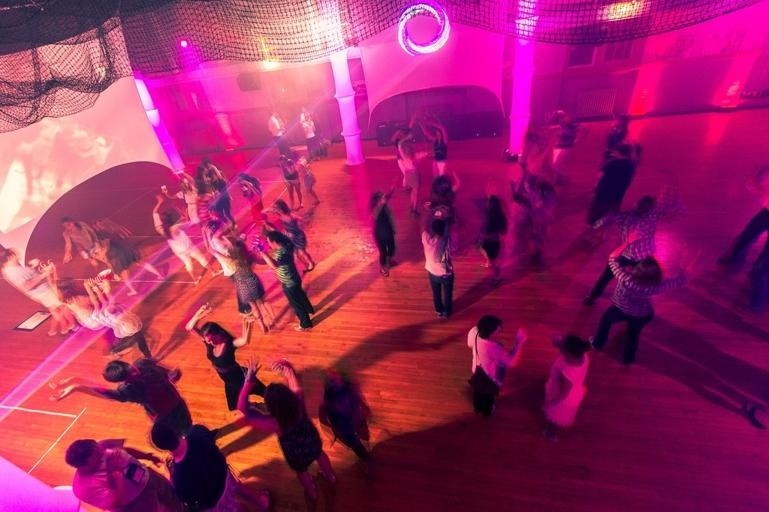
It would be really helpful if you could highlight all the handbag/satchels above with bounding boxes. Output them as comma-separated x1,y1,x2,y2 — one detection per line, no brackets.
465,366,499,413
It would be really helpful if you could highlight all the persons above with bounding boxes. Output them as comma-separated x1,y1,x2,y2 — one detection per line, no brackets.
369,111,662,440
267,110,322,161
151,154,320,332
2,218,153,359
51,303,377,512
